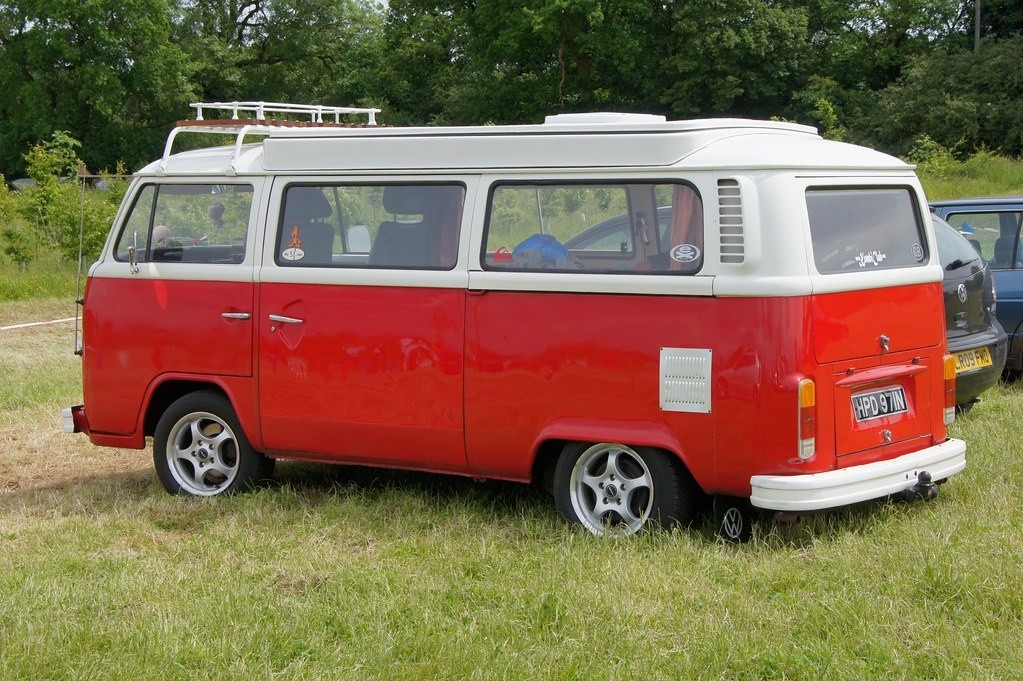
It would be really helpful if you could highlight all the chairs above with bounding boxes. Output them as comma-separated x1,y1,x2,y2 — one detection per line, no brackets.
990,234,1023,267
366,185,443,267
280,187,335,265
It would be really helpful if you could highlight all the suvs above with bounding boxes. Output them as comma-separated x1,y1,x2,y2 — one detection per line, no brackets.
557,207,1009,418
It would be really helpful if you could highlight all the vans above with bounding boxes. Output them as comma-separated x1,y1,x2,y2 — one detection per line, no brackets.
72,100,967,543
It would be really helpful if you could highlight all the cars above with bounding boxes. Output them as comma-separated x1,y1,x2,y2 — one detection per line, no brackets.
928,197,1023,372
